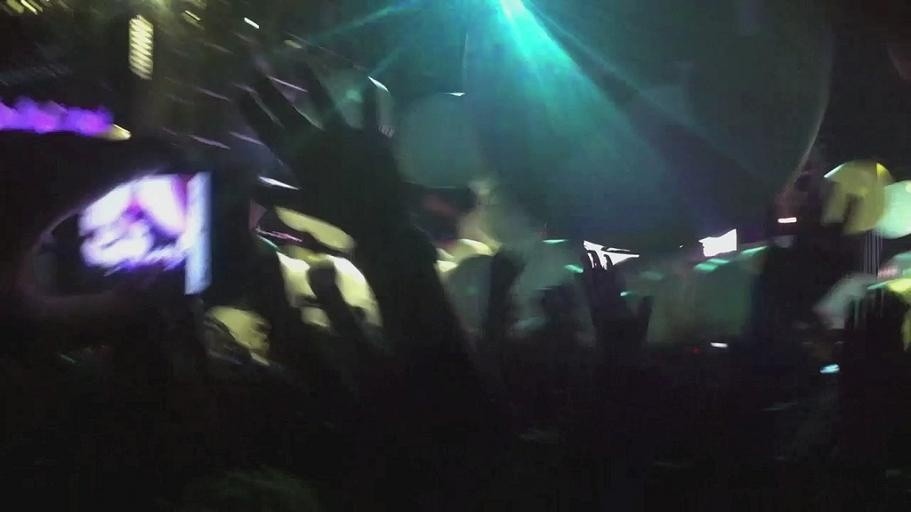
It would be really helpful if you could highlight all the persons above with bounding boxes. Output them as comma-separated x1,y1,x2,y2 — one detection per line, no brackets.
1,58,911,511
